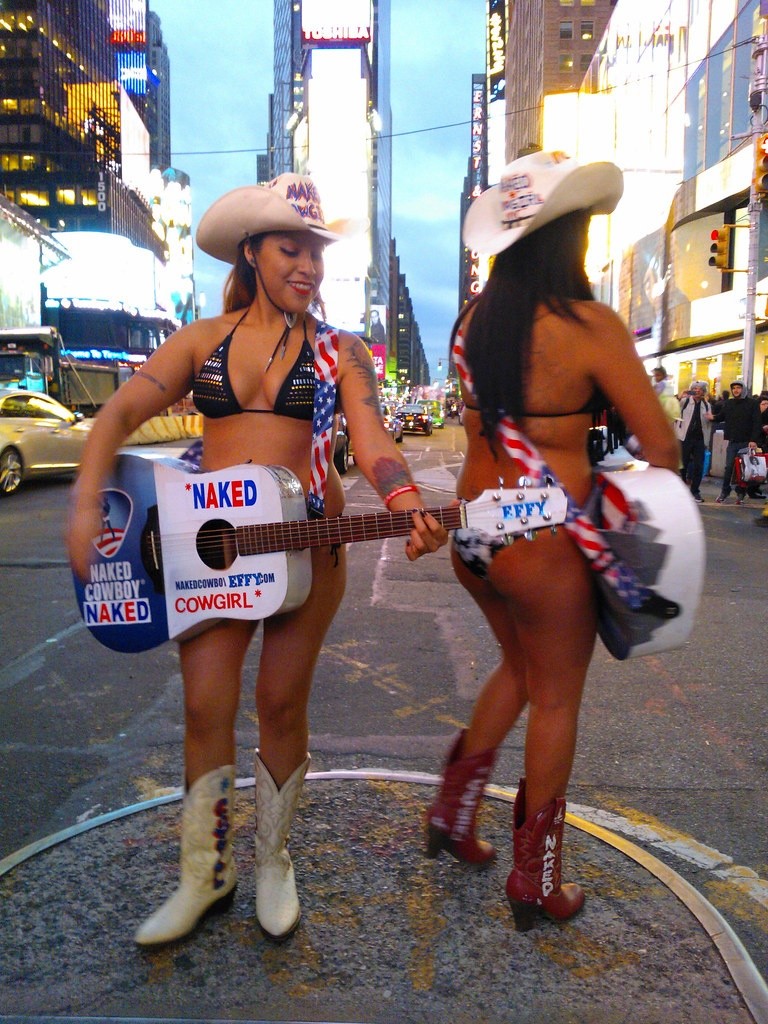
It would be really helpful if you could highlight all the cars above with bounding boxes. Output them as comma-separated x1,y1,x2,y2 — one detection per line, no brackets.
393,403,434,437
380,402,403,443
334,412,349,477
0,387,96,498
379,398,407,417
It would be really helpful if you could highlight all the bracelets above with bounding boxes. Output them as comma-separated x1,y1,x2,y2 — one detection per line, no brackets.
382,484,419,510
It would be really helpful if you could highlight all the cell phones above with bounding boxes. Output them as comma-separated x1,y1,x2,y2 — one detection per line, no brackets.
686,391,696,395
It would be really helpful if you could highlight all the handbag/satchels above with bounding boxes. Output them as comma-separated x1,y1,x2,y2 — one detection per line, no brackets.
729,445,768,489
701,449,711,478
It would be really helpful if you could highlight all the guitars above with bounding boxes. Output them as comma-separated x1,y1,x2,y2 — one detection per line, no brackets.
68,450,570,658
587,421,708,662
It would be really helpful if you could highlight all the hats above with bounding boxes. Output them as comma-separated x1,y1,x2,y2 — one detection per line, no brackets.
653,366,665,373
195,173,371,264
691,382,705,391
463,148,625,256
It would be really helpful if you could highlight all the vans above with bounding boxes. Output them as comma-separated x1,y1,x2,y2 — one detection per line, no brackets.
415,399,447,429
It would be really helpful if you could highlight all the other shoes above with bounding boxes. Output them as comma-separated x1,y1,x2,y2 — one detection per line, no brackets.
691,490,705,503
749,486,763,495
716,492,730,503
747,491,765,499
735,494,745,505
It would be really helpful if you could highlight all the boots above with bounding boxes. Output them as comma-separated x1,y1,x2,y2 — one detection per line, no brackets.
426,728,500,867
506,777,584,932
254,748,310,943
132,763,237,949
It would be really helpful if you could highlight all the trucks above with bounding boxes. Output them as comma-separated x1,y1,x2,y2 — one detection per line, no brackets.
0,328,119,417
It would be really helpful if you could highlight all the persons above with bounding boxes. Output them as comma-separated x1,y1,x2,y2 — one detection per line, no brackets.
420,150,683,928
61,171,462,953
650,365,768,527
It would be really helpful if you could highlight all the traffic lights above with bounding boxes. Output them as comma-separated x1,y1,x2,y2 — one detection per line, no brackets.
708,225,730,269
754,133,768,204
